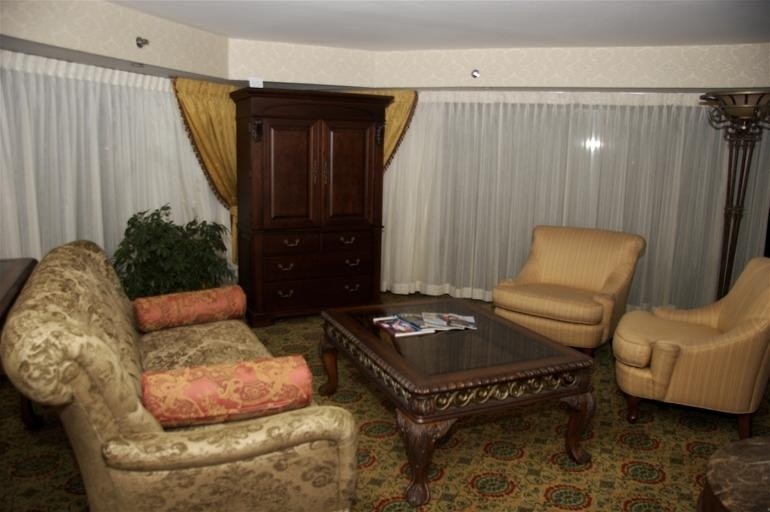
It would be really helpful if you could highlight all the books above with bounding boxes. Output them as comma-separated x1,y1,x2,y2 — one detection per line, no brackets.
371,310,479,338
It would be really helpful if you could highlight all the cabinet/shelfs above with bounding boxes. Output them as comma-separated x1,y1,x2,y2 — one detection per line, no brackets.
230,85,396,328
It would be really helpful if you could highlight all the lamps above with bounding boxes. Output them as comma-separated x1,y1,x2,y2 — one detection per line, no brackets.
700,87,770,302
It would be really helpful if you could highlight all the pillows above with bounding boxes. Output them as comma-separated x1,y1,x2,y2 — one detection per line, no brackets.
141,353,314,429
133,282,247,332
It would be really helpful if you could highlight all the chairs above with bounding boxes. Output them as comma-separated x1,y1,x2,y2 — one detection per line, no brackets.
611,257,770,416
492,224,645,349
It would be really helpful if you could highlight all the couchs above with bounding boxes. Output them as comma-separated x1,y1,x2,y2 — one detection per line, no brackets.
0,239,359,512
